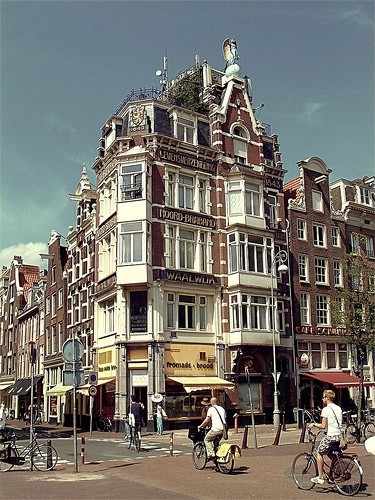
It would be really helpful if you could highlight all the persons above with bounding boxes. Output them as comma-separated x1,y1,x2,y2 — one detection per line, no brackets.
0,401,7,431
153,403,164,435
201,398,212,428
197,397,227,462
125,396,142,442
307,390,343,485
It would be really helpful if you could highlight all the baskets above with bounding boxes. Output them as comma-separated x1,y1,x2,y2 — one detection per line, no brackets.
188,427,205,441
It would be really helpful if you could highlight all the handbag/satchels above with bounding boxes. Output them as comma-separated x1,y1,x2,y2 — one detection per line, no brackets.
216,443,243,463
128,413,136,427
223,426,229,440
339,431,348,450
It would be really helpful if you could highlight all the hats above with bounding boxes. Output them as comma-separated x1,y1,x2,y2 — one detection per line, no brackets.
201,398,210,406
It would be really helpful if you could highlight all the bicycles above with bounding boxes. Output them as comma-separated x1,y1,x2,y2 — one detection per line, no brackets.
344,410,375,444
94,410,113,433
291,426,365,496
0,427,58,472
303,405,321,424
188,426,242,474
123,416,142,453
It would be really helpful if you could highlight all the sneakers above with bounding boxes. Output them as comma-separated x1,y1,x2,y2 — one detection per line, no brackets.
311,477,324,485
125,434,130,440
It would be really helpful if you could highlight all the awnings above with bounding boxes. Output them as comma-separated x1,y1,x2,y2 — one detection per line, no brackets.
169,376,236,393
46,382,77,398
74,379,115,396
5,376,45,397
300,371,375,389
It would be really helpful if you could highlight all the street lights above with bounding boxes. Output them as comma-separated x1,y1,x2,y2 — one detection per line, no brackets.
270,250,289,431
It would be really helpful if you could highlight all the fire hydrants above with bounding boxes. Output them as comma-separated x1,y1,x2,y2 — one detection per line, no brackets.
233,413,239,433
